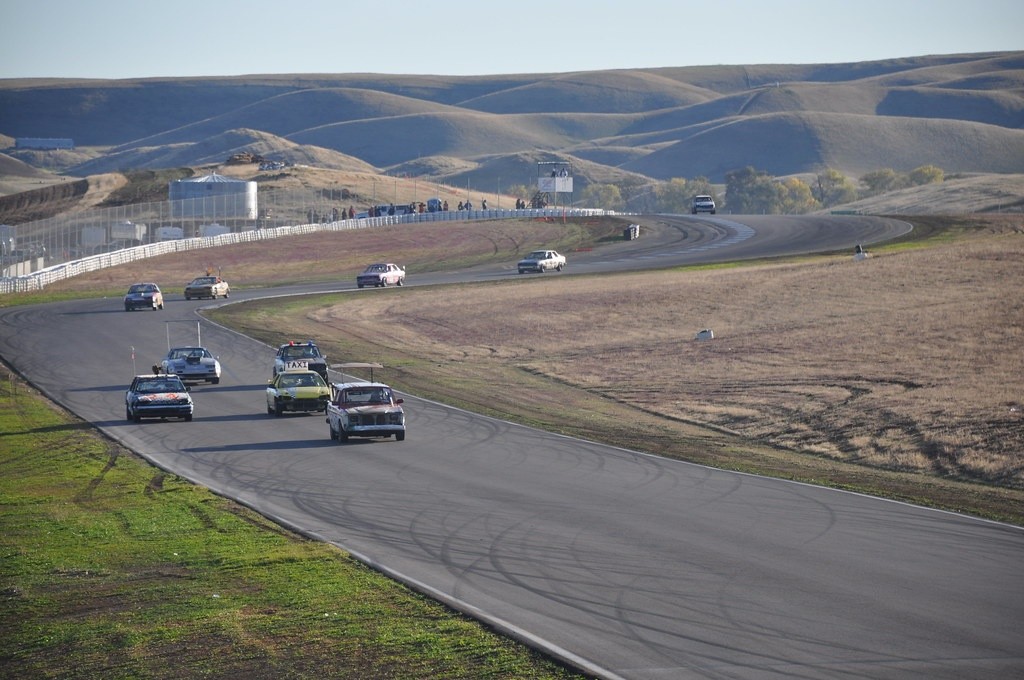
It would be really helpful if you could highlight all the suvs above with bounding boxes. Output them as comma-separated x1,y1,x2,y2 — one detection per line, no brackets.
356,204,409,221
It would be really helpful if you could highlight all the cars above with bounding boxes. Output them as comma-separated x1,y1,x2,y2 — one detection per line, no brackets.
124,283,164,312
517,249,567,274
356,262,406,287
266,360,331,417
326,381,406,444
184,276,232,299
274,339,329,385
161,344,221,385
125,363,194,423
691,194,716,215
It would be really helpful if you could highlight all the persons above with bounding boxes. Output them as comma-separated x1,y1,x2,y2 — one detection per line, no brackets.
197,351,205,358
373,390,387,403
307,168,569,224
301,375,319,386
301,347,316,358
